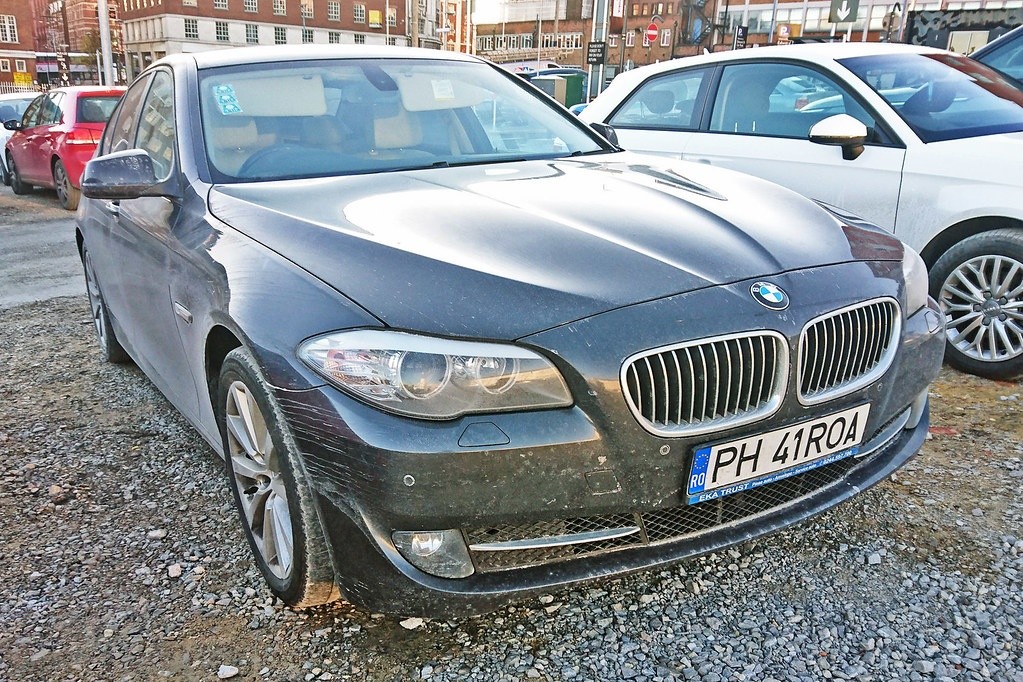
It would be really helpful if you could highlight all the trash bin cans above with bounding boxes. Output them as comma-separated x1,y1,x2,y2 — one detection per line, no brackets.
559,74,585,107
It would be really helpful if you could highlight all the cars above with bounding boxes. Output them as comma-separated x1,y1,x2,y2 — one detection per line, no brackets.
0,28,1023,380
75,45,943,620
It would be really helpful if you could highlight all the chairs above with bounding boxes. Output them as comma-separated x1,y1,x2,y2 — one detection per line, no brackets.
213,104,261,177
83,101,105,122
641,90,678,126
0,105,21,122
357,99,434,158
727,81,771,132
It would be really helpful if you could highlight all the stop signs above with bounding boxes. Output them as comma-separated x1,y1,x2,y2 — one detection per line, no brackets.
647,24,658,41
